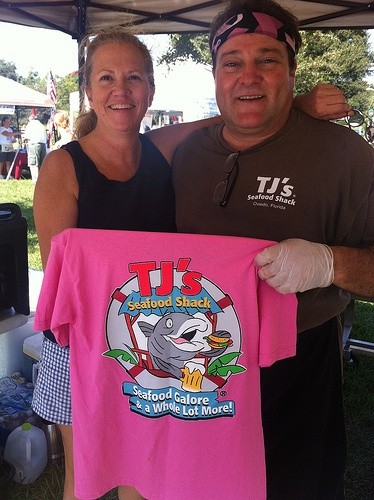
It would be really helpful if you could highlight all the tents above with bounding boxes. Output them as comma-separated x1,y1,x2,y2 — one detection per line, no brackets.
0,74,55,176
66,76,191,144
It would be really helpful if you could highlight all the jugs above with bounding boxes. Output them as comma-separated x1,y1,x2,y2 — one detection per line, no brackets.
38,417,62,459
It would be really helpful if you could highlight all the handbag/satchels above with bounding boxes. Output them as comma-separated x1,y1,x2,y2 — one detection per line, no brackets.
2,143,13,152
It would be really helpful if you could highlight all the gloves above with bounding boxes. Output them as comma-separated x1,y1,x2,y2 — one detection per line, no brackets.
253,238,334,294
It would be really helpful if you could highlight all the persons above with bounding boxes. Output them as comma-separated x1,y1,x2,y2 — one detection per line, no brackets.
25,115,47,181
0,117,21,179
46,111,80,152
32,26,354,500
172,0,374,500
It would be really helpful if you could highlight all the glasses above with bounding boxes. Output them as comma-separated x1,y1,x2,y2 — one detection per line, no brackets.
344,109,374,144
212,151,241,208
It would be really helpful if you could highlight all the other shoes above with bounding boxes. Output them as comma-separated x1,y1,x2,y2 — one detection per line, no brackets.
0,175,5,179
7,175,14,180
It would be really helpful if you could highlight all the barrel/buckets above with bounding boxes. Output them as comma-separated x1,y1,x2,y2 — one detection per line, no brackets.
5,423,48,484
